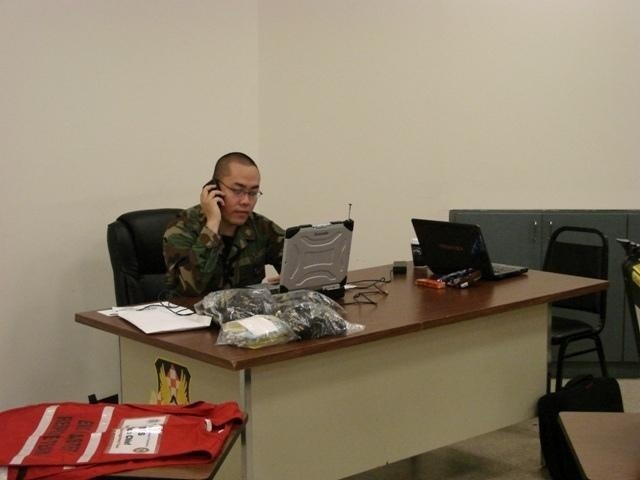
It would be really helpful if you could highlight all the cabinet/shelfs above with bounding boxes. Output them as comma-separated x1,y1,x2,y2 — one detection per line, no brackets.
448,209,640,378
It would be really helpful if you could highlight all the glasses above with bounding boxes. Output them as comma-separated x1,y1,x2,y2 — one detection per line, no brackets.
219,179,262,200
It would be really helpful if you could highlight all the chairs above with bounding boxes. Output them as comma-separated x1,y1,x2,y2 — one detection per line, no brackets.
541,225,610,394
106,207,190,307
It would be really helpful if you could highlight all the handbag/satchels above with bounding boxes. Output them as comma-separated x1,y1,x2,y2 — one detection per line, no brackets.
536,374,622,479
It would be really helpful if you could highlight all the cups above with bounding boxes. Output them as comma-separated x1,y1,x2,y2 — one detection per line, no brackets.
411,239,427,266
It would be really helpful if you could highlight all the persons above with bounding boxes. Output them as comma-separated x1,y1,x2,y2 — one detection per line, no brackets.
162,153,287,301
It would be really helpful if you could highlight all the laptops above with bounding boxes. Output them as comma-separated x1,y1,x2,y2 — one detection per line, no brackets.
231,219,353,299
411,218,528,280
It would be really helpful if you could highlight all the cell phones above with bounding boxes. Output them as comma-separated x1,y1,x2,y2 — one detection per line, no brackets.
203,180,223,211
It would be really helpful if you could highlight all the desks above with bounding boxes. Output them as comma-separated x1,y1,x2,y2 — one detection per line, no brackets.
74,261,610,480
